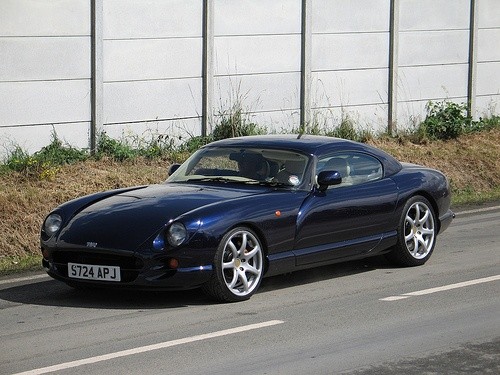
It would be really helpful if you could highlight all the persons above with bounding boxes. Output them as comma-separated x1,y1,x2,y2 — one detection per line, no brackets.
237,157,261,180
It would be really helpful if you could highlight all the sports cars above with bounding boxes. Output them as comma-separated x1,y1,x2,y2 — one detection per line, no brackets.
40,133,455,302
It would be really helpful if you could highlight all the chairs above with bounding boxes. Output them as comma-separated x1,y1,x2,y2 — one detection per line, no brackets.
236,156,354,190
